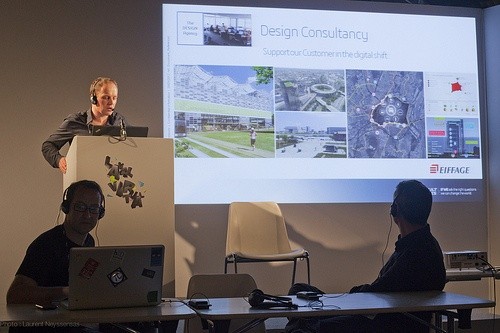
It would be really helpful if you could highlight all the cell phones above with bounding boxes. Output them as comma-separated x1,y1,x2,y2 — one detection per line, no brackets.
35,303,58,309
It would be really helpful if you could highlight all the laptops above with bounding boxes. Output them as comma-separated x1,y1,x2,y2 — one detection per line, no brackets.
59,244,165,309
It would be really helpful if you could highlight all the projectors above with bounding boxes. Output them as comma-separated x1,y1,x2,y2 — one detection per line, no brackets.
443,251,489,270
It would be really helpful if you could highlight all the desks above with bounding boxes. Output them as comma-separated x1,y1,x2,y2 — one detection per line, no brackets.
0,292,496,333
446,266,500,281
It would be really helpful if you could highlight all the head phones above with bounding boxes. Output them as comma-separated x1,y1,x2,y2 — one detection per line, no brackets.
89,78,100,104
248,289,292,307
390,185,405,217
60,184,106,220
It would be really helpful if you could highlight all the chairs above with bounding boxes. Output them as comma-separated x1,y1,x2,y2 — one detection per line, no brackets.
224,201,310,284
187,274,257,298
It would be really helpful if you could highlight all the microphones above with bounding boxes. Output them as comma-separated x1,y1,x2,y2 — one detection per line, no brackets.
112,109,125,137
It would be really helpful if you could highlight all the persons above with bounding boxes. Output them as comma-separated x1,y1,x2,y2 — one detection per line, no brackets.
41,76,130,174
210,25,215,31
249,127,257,151
6,179,106,333
216,25,221,34
285,179,447,333
